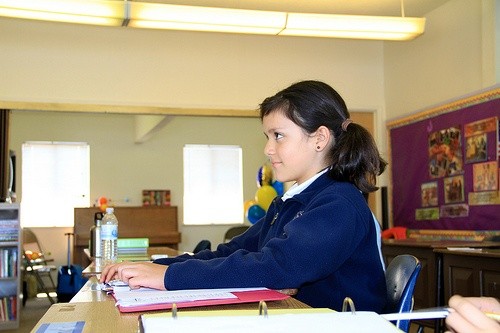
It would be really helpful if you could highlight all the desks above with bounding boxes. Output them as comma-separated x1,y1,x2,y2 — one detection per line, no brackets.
382,238,500,333
31,247,312,333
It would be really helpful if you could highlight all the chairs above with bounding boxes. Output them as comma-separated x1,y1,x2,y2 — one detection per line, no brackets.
382,255,421,332
23,228,56,305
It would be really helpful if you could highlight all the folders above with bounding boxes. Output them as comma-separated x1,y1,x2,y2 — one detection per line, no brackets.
107,279,290,313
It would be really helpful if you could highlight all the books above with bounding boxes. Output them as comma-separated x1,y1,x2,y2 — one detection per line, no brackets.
0,249,17,279
0,295,17,322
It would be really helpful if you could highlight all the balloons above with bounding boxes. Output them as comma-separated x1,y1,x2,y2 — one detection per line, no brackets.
243,164,284,224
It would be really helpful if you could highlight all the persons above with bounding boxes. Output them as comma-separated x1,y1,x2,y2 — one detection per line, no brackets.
99,81,396,316
443,295,500,333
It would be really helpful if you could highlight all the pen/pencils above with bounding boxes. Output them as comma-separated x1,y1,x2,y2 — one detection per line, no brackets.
443,307,500,323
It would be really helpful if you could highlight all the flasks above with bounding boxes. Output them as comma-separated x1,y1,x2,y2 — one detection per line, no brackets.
89,211,104,258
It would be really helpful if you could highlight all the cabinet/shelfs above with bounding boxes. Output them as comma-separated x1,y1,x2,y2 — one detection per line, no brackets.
0,204,22,330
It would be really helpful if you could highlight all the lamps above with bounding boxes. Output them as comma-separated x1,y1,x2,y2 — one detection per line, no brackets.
0,0,425,41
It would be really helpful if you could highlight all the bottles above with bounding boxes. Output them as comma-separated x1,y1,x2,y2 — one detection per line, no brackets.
100,208,118,262
